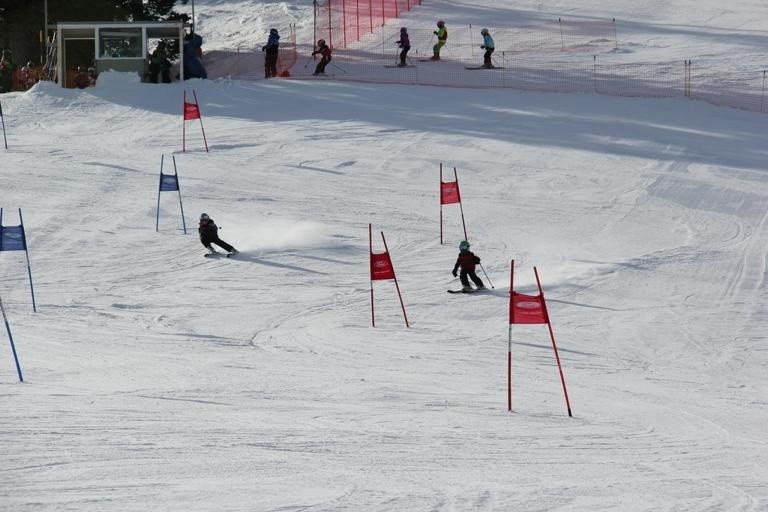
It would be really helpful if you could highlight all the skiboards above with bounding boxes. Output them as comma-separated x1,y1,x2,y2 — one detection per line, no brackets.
203,252,239,259
385,64,417,68
466,63,505,70
446,286,489,294
420,58,448,62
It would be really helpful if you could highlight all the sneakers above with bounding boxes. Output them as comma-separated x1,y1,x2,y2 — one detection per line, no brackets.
463,284,485,291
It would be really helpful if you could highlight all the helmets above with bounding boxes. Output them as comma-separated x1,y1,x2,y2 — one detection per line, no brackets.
269,28,278,34
437,21,445,28
459,241,470,252
318,39,325,47
481,28,488,35
200,214,210,223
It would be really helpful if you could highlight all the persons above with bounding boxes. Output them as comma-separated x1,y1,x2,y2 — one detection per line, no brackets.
451,240,484,291
430,20,448,61
150,41,166,83
198,212,239,255
394,27,410,65
311,38,332,75
480,28,494,66
261,28,280,78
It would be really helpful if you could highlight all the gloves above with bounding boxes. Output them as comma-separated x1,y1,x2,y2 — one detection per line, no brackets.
452,269,458,277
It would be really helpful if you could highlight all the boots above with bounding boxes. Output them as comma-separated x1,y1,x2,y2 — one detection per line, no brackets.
209,248,238,254
